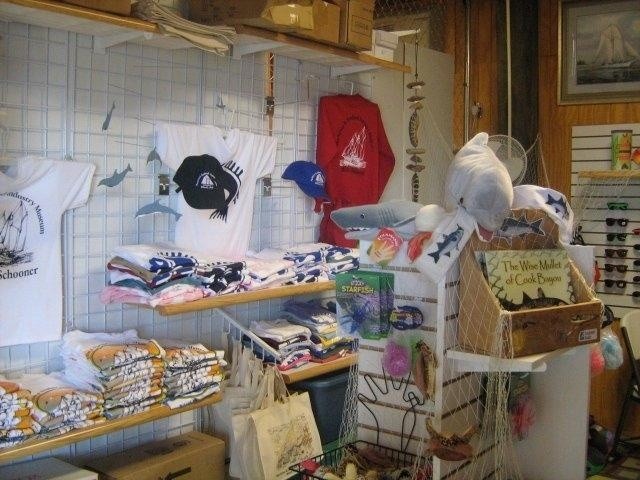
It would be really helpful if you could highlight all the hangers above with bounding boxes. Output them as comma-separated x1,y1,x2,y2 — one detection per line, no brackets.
0,124,17,163
219,106,237,132
342,80,358,97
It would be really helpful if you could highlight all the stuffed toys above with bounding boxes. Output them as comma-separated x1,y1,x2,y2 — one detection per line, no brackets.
326,196,433,239
414,131,515,238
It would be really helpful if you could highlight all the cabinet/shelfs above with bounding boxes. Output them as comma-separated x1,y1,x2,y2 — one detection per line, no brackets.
124,281,356,383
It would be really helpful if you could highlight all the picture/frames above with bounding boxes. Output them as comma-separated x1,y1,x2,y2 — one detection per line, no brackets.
557,1,639,107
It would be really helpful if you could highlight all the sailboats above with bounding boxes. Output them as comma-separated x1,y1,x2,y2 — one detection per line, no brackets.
576,22,640,80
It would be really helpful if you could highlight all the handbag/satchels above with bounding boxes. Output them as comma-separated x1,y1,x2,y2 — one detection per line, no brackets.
204,332,325,480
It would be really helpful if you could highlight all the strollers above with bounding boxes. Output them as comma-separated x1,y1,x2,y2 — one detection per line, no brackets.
589,304,640,465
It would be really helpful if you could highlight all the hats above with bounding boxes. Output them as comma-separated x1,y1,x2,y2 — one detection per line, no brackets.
281,161,331,202
173,154,227,210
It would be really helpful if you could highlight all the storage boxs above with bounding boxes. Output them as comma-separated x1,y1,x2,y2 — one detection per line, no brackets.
0,453,101,480
293,370,356,445
189,0,340,46
330,0,374,52
88,429,225,480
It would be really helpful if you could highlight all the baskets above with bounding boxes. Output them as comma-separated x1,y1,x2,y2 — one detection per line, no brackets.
288,439,427,479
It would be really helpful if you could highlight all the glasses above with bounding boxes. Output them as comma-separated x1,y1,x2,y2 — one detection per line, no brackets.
605,203,640,297
